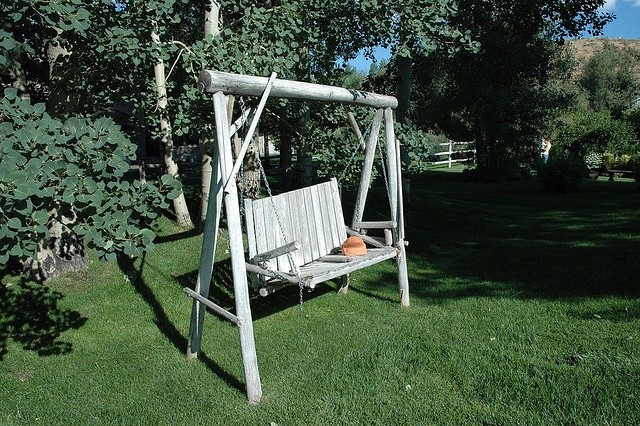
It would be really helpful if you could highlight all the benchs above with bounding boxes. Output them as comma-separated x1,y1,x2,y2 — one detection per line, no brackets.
243,175,402,298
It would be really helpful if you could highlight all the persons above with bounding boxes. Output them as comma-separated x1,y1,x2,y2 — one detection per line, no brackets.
540,138,551,163
